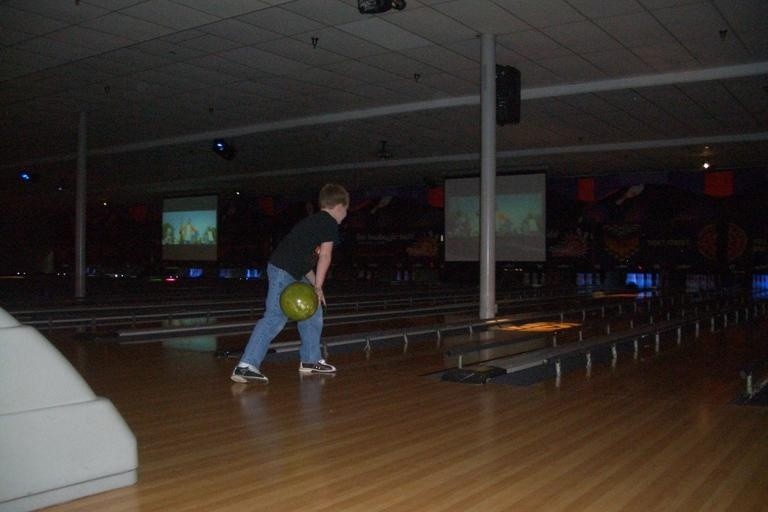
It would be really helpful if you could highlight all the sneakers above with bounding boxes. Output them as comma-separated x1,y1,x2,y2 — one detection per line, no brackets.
298,360,337,373
230,366,269,384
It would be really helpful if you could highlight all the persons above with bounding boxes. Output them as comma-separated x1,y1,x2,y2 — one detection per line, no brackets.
230,181,350,383
178,217,197,245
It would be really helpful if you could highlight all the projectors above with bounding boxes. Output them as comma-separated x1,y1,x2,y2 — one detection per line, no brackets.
377,151,392,158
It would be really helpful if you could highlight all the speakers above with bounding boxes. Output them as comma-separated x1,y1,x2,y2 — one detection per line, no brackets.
496,64,520,125
